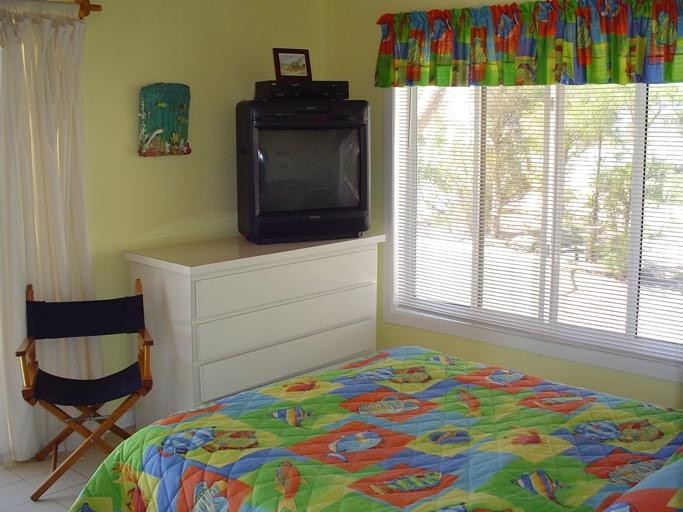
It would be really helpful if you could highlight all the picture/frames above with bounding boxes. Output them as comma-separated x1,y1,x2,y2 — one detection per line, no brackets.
273,48,312,80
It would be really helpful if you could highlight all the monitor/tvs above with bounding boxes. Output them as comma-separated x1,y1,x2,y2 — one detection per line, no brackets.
234,99,372,245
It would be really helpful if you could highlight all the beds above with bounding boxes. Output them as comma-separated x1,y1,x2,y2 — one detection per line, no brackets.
69,347,683,512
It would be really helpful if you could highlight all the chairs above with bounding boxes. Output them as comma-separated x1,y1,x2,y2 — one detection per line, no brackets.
16,279,153,501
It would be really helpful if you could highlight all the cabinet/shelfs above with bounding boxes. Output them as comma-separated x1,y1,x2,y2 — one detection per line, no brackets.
122,233,386,434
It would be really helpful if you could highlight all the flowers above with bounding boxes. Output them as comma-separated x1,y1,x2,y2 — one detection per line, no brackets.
157,102,168,146
